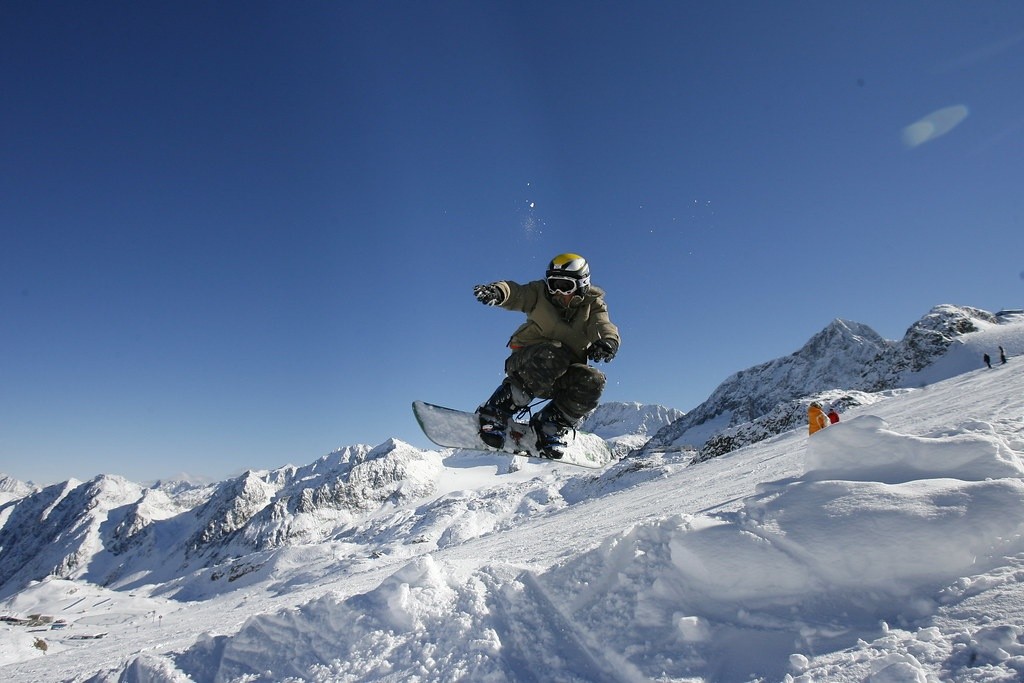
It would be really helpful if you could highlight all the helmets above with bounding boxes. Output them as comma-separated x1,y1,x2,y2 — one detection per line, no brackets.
546,253,590,296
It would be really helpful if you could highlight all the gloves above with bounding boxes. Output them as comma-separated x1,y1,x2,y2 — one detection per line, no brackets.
474,284,503,307
582,338,618,363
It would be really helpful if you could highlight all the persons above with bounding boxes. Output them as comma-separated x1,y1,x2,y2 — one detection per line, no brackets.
984,353,992,369
808,401,839,435
471,254,620,459
999,345,1006,364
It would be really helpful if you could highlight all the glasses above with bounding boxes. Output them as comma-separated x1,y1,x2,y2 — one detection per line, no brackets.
547,275,591,295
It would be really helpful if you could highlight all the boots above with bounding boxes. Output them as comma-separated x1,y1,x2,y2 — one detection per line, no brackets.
474,377,528,449
528,397,585,460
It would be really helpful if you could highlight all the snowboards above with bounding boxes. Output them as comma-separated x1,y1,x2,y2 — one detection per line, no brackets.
411,400,611,468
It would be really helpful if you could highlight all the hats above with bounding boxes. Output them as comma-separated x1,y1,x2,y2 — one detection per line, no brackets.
810,402,821,408
